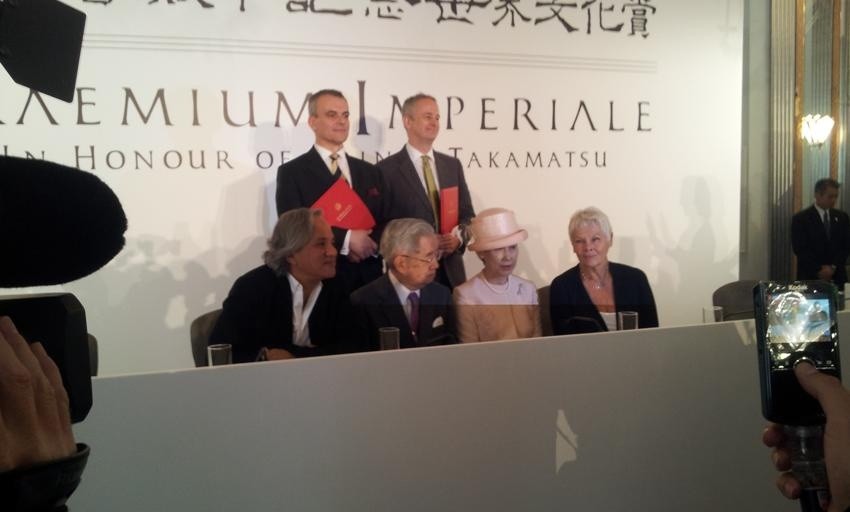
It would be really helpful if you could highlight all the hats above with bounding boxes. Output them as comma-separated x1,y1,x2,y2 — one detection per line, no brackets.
468,209,529,253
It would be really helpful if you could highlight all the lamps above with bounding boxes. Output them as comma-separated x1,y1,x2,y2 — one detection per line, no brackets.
803,114,835,145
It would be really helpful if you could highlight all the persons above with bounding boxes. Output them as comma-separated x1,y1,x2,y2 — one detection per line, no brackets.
374,96,476,294
359,217,459,352
205,208,360,366
809,302,828,322
762,360,850,512
0,316,90,512
550,207,659,336
453,208,542,343
276,89,384,293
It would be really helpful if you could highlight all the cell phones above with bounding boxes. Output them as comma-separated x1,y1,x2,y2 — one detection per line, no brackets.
753,281,842,424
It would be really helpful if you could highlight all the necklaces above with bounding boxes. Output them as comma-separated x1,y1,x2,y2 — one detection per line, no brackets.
480,271,512,294
580,268,608,288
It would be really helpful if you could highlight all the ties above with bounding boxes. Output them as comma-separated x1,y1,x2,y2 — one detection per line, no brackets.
332,152,351,187
422,155,443,234
408,292,419,338
824,208,833,237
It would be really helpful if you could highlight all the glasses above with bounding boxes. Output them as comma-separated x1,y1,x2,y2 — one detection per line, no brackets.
403,250,442,263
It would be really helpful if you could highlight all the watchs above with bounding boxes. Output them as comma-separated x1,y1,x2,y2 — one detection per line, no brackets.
832,264,837,273
791,179,850,291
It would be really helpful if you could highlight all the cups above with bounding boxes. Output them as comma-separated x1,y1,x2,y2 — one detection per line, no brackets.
618,312,639,331
207,342,234,367
703,306,723,321
379,327,400,351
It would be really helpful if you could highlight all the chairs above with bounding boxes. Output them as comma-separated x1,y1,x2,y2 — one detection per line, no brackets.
537,285,558,336
712,278,760,323
191,308,221,367
